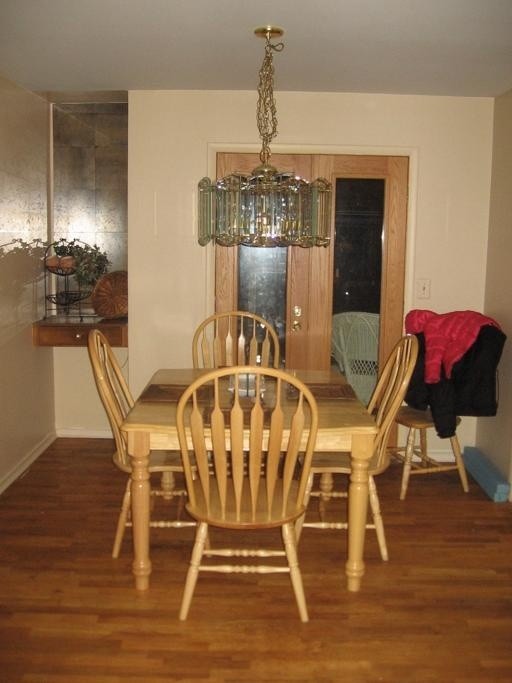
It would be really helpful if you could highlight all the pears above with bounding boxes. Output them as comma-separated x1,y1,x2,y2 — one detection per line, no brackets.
45,256,76,272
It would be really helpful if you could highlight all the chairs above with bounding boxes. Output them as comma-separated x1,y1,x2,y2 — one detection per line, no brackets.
296,333,418,561
176,365,308,622
87,328,210,557
191,310,280,371
330,312,380,408
388,405,469,501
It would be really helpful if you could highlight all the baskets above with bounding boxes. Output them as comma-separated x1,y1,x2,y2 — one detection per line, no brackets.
46,291,91,305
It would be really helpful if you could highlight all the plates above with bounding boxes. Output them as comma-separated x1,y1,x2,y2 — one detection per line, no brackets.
226,374,266,395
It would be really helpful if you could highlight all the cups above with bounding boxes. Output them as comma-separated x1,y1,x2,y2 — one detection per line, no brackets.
286,383,298,400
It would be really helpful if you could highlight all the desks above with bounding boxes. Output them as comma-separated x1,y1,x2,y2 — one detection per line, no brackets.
120,368,379,591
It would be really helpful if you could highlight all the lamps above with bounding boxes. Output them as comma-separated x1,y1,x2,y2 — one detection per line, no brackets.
196,26,331,247
